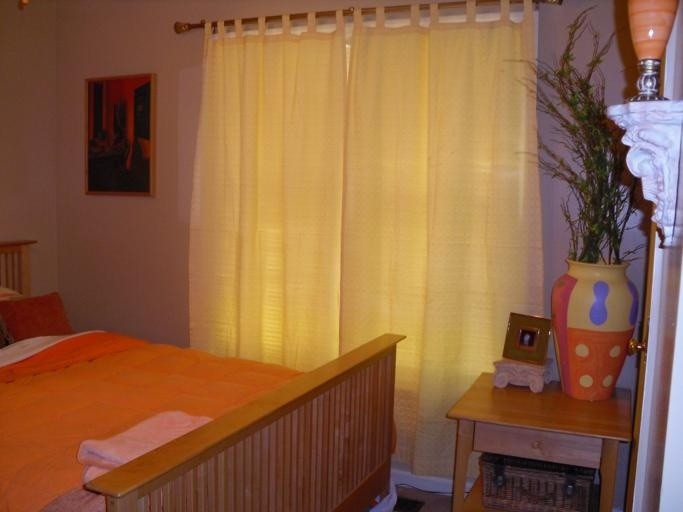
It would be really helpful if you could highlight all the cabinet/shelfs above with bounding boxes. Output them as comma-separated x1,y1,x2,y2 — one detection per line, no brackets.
447,371,634,512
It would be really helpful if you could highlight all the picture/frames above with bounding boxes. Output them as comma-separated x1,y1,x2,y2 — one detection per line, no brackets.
86,73,157,195
501,312,552,368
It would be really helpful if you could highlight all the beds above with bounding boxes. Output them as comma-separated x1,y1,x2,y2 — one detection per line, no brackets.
1,238,408,510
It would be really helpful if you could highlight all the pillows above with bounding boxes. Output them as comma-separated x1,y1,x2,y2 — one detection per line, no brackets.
1,292,72,344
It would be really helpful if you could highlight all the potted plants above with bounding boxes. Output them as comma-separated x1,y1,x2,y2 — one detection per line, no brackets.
505,4,638,403
